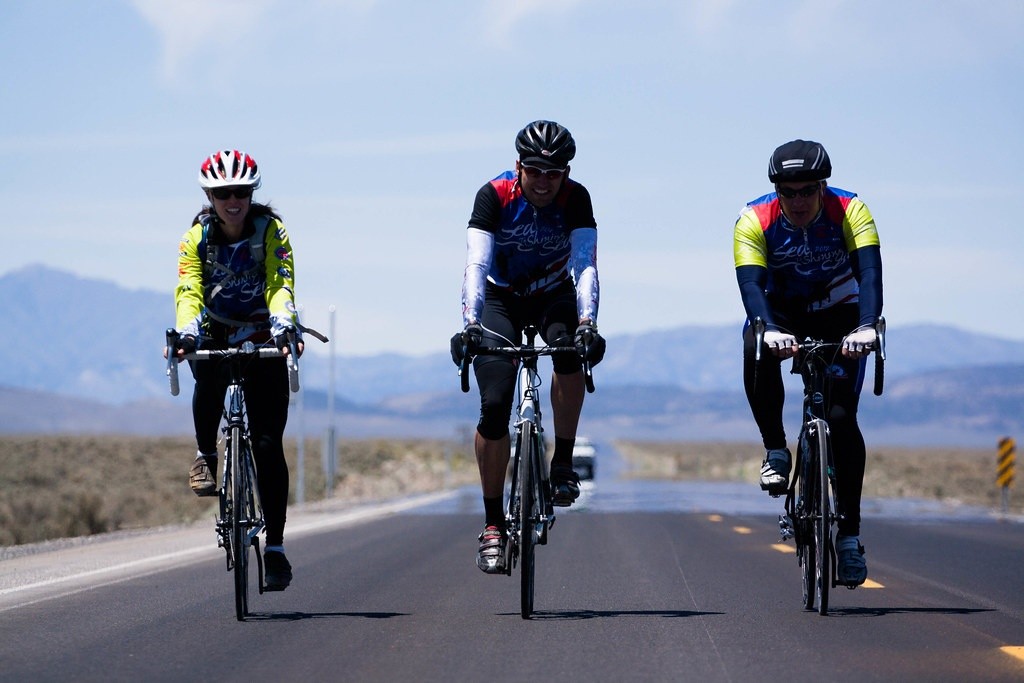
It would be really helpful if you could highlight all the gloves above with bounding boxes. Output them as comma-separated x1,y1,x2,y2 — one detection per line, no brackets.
450,324,484,365
574,324,606,368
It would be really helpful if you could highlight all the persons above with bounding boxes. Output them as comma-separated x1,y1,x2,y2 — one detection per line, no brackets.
450,120,606,573
164,151,305,587
733,139,884,586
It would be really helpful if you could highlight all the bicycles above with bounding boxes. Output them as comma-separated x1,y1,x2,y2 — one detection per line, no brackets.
752,316,887,615
459,323,595,620
166,322,299,622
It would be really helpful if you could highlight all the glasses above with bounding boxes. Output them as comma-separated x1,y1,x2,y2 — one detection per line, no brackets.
211,185,252,200
521,162,568,180
776,182,821,198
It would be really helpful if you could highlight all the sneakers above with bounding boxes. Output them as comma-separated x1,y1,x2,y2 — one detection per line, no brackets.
475,524,508,571
550,465,580,498
835,530,868,585
189,453,218,494
264,551,292,587
760,448,793,491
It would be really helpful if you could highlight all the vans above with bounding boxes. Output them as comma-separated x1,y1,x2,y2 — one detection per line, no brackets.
573,445,598,492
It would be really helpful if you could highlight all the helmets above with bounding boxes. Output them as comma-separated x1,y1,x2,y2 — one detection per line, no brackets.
199,149,261,191
769,139,831,184
516,120,576,167
